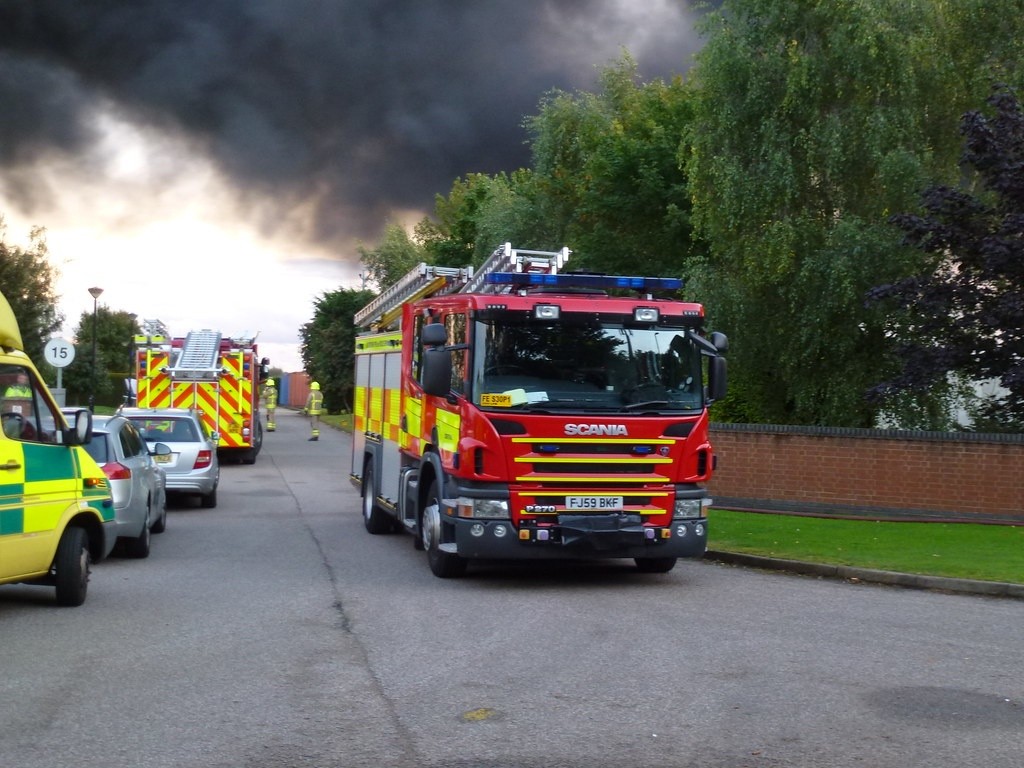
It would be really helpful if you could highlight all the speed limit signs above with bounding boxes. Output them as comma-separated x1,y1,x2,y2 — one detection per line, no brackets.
44,337,76,368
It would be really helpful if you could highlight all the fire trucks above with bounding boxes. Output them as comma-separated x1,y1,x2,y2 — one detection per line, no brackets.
349,242,732,575
129,316,271,465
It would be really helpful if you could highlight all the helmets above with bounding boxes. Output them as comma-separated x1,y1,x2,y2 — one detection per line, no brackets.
310,382,320,390
266,379,275,385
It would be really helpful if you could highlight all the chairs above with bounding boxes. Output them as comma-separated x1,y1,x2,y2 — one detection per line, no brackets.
498,329,538,374
172,421,189,440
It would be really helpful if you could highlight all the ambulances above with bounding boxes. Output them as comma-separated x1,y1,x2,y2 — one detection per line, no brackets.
0,289,122,608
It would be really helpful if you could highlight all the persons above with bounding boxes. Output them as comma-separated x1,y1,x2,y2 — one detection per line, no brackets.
263,379,277,432
6,374,32,397
305,382,323,442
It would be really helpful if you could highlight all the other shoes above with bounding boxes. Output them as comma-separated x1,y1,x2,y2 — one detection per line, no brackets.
267,429,274,432
309,436,318,441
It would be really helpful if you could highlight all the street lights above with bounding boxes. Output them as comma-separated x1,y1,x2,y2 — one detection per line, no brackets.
127,312,137,404
87,286,103,414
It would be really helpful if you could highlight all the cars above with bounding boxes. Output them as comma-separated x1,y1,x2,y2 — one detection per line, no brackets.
115,403,221,508
36,412,172,559
